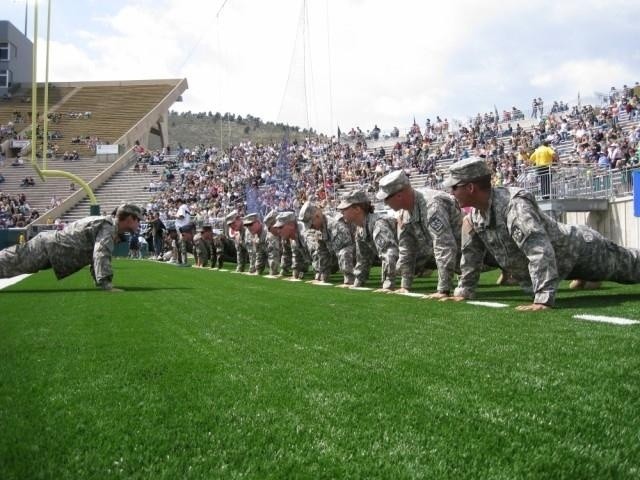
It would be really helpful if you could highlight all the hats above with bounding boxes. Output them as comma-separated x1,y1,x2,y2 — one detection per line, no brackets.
337,189,370,210
441,155,492,188
117,203,145,221
165,200,317,234
375,169,410,200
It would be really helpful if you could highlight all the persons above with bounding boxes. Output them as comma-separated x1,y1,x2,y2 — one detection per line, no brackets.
0,203,143,293
127,191,515,291
435,157,640,309
0,91,93,233
376,170,601,300
131,81,640,213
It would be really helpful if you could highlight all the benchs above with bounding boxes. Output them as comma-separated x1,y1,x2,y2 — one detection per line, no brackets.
332,93,639,211
1,79,189,157
0,155,166,232
